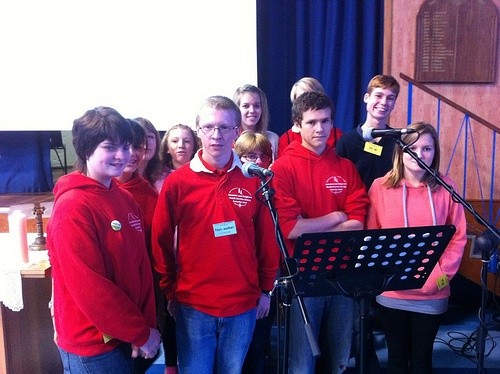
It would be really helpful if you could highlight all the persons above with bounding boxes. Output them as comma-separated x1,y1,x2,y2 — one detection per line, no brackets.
266,91,370,374
366,122,466,374
336,75,401,193
230,77,343,168
112,116,199,374
150,96,280,374
46,106,161,374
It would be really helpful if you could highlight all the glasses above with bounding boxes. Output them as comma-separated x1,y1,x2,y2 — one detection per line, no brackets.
242,155,270,163
197,123,239,134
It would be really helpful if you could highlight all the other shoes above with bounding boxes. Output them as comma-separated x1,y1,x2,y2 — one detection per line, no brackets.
165,367,178,374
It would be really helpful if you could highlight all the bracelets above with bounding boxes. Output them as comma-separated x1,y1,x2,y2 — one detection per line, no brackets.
262,293,271,299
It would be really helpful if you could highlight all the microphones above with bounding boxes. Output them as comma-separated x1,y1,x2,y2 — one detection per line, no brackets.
363,127,416,141
242,162,273,178
